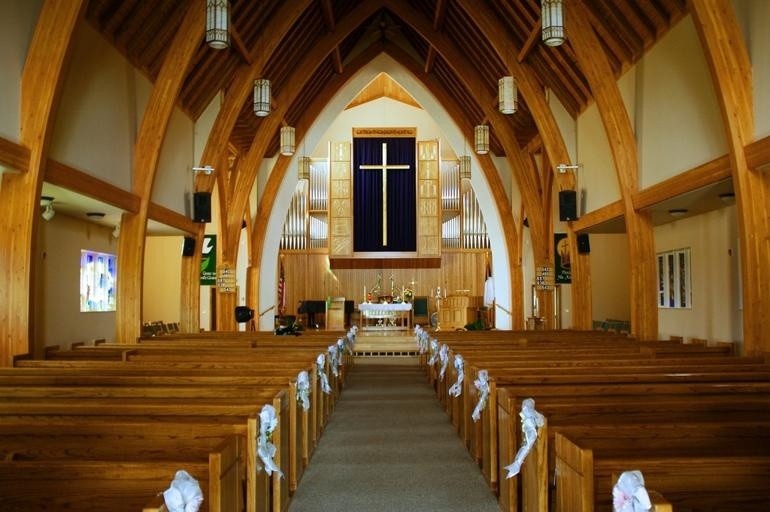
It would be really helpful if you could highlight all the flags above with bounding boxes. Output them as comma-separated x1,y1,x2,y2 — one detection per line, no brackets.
278,260,287,319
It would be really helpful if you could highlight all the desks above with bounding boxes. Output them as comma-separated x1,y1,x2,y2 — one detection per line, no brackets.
358,304,412,331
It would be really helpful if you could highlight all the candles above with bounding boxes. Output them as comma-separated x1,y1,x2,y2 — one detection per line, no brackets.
364,285,366,303
402,285,404,301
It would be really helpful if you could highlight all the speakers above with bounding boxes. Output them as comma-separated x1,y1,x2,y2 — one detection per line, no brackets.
194,191,212,224
579,234,591,252
558,190,578,222
182,237,196,255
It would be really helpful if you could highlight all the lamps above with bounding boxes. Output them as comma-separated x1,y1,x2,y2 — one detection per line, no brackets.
206,0,231,49
254,79,272,117
460,156,471,182
298,157,310,181
40,196,56,221
474,125,489,155
540,0,566,47
498,76,518,114
280,126,296,156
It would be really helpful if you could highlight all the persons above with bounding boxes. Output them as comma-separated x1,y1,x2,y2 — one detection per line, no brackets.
274,316,296,336
463,309,494,331
292,313,308,332
